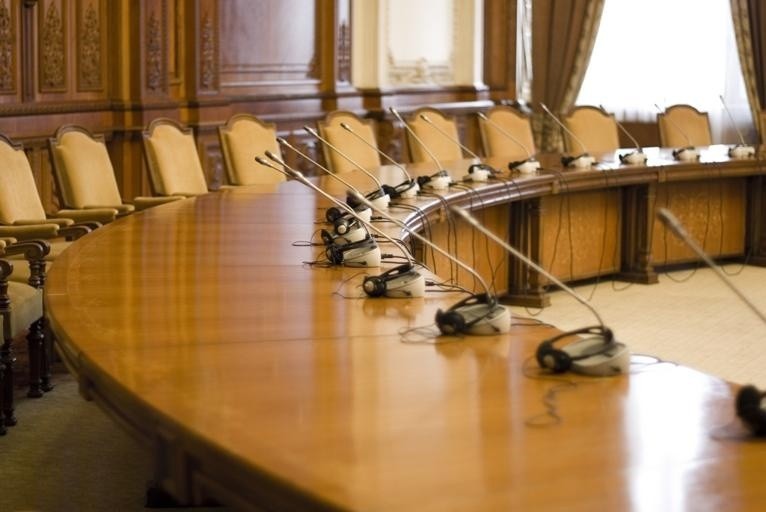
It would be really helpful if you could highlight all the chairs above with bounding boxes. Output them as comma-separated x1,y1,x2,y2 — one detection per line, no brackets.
0,133,87,436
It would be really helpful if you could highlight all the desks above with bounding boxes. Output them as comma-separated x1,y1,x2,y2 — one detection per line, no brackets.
41,140,765,512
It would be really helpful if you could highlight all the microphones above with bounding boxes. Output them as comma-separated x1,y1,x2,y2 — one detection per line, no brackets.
255,156,371,246
346,188,511,335
287,169,426,298
265,149,381,268
657,208,766,324
451,204,629,377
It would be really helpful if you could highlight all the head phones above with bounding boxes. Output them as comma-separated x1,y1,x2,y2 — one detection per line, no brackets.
363,261,414,297
435,293,498,335
320,216,357,243
536,326,616,374
737,386,766,438
325,235,371,264
